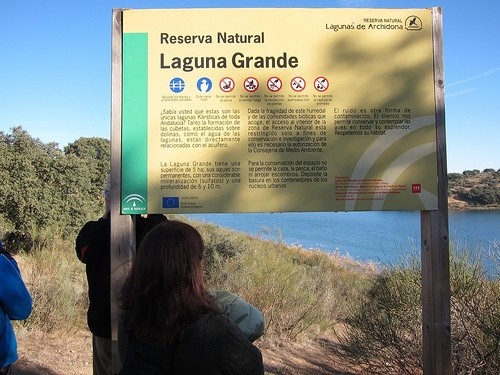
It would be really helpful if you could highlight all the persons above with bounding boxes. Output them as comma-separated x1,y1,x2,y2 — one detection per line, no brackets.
0,242,33,375
77,173,170,375
118,219,265,375
